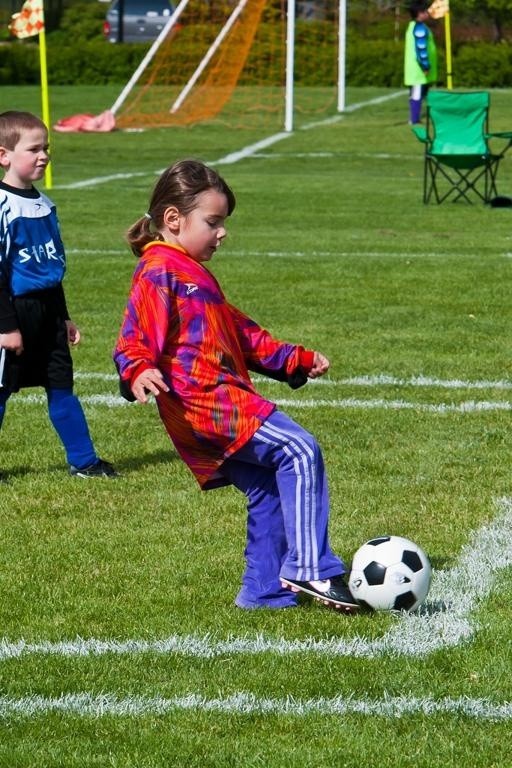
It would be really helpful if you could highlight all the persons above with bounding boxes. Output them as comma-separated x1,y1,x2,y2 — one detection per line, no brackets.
0,110,118,478
111,161,366,614
398,1,440,142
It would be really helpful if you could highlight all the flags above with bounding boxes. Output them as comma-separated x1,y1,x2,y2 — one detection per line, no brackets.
426,0,451,21
8,1,44,47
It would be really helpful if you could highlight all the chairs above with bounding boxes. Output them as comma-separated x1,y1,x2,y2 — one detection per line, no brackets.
410,89,511,205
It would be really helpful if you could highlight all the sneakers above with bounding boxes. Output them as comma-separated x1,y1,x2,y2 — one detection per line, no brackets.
70,460,126,480
280,575,360,613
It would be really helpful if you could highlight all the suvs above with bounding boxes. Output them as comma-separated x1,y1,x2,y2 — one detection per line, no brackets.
101,0,181,45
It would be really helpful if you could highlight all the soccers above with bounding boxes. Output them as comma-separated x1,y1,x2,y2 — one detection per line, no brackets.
348,537,434,613
